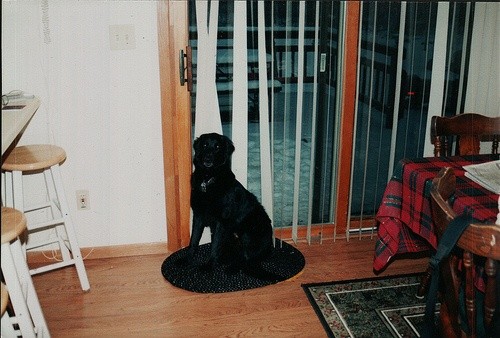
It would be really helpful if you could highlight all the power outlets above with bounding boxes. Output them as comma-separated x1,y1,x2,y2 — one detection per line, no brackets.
75,189,90,211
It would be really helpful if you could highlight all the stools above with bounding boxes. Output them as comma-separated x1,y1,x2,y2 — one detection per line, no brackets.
0,144,91,291
0,206,52,338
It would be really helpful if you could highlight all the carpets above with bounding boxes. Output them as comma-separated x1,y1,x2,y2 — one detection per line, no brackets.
301,272,441,338
160,236,305,294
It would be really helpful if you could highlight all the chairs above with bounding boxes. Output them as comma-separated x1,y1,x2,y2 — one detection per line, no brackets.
430,165,500,338
430,113,500,157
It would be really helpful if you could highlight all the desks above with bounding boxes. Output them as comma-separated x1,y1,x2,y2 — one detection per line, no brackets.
191,47,271,111
372,153,500,299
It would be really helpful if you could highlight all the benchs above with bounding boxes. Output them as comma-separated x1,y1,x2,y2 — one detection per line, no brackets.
192,80,282,123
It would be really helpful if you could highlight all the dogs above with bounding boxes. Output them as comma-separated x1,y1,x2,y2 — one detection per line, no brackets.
185,131,286,285
